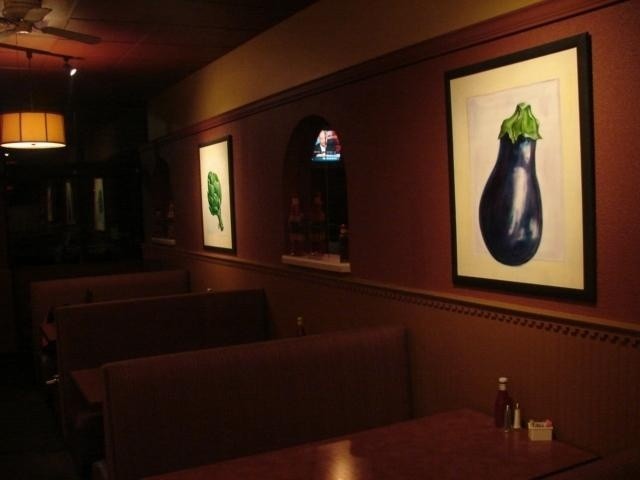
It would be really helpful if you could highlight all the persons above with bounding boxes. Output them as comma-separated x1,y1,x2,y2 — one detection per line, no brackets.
326,130,337,141
315,130,326,153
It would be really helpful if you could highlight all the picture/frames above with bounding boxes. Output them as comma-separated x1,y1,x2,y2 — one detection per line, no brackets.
442,31,592,300
194,131,238,256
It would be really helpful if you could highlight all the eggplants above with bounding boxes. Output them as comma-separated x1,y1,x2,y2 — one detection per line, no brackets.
480,105,543,265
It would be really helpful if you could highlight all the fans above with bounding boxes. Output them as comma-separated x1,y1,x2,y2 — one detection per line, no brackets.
1,0,101,50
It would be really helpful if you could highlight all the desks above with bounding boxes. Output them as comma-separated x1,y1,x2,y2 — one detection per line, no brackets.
161,406,602,479
70,368,104,408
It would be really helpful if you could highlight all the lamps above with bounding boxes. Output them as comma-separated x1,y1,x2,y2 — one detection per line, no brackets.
0,52,66,154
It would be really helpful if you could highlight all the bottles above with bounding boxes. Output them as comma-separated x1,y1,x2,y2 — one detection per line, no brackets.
494,376,513,428
288,190,349,262
514,403,522,430
295,315,306,336
504,404,512,431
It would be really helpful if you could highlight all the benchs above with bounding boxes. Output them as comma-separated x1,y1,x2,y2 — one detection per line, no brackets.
18,254,638,479
